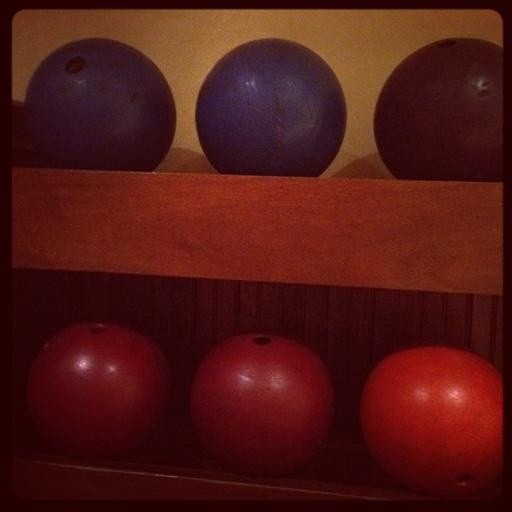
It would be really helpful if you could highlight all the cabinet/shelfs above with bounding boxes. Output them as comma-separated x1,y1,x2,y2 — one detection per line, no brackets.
0,162,507,508
